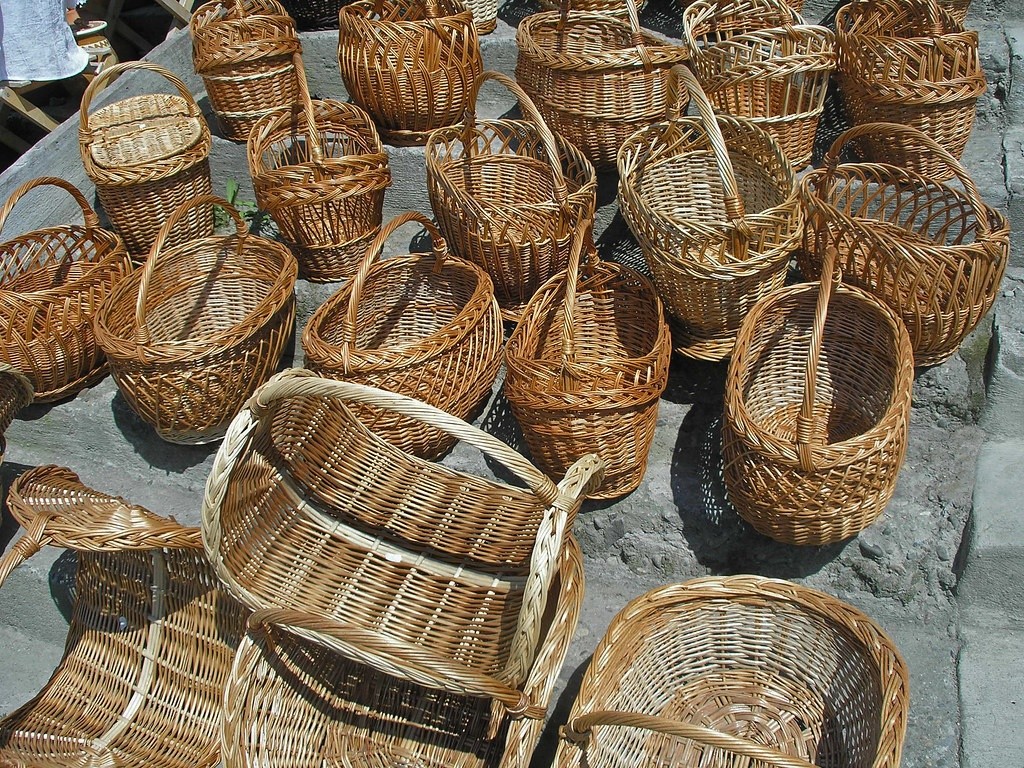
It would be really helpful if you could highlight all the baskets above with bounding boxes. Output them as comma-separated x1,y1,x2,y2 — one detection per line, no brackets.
720,246,914,546
617,64,804,364
219,533,585,768
503,218,672,502
0,177,135,404
796,122,1011,367
79,61,215,267
0,463,253,768
550,574,910,768
0,362,36,456
247,51,393,283
337,0,484,148
462,0,498,35
514,0,988,184
200,367,607,699
93,195,299,446
425,71,598,324
300,210,503,463
188,0,304,142
